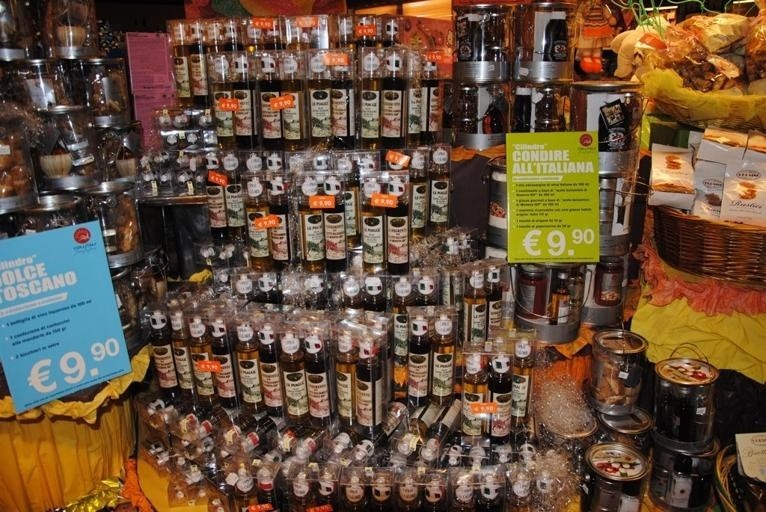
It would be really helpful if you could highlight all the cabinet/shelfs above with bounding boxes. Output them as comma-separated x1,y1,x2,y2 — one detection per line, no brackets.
135,182,293,289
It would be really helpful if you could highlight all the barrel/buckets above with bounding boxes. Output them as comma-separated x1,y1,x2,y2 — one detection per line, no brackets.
572,78,650,174
507,260,593,343
586,317,649,416
582,441,652,512
539,413,598,452
457,81,511,154
582,252,629,325
512,2,580,81
649,433,720,512
512,78,572,133
483,156,508,249
453,3,514,81
598,176,654,256
596,408,651,443
653,341,720,444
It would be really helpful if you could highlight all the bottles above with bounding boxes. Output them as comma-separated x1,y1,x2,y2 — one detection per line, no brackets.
146,393,172,418
404,51,422,151
331,430,350,457
511,87,533,136
420,399,461,462
351,399,408,463
88,58,130,128
398,401,444,457
384,178,410,275
233,325,266,415
146,443,279,512
236,273,255,303
356,336,383,437
255,275,279,305
39,1,99,61
534,87,559,132
72,181,144,269
307,53,331,152
304,277,327,311
158,397,193,427
485,354,515,445
382,51,407,150
393,277,416,316
113,269,142,355
323,174,346,275
222,153,249,244
277,421,305,455
407,314,431,407
432,313,456,408
409,150,428,239
168,309,199,405
190,22,209,112
188,315,216,410
280,460,426,511
487,266,503,345
232,54,258,151
149,307,181,403
270,176,299,273
459,87,475,134
441,239,465,311
331,53,357,151
281,56,307,152
179,405,216,435
223,410,267,446
360,178,389,275
338,156,363,249
304,335,334,429
210,320,237,411
426,440,553,511
192,409,237,440
294,419,343,466
462,272,487,348
206,23,224,56
516,264,546,322
1,0,29,62
358,51,384,150
417,275,437,308
513,338,533,430
212,55,236,151
0,110,41,215
594,255,626,307
258,325,284,418
19,191,90,233
279,331,311,427
549,268,572,325
247,179,272,274
220,19,404,61
340,278,362,310
258,55,282,151
429,146,450,236
51,105,103,169
363,277,387,313
239,414,286,455
334,335,358,429
27,59,63,111
299,176,326,274
203,154,229,245
461,353,487,438
171,22,191,110
422,60,442,144
